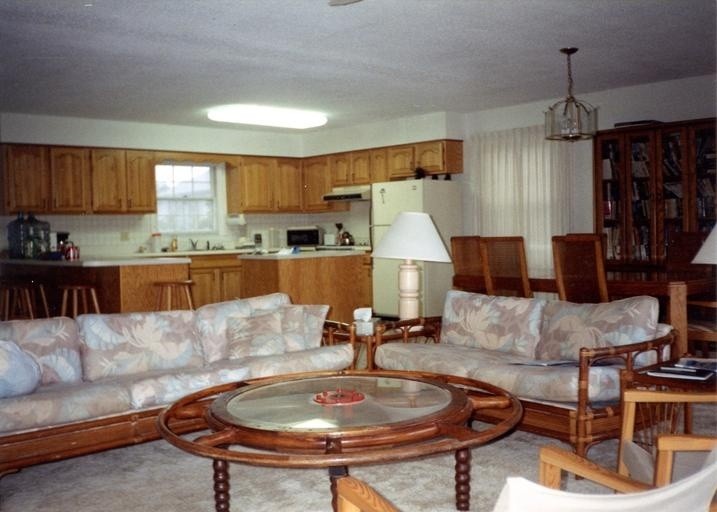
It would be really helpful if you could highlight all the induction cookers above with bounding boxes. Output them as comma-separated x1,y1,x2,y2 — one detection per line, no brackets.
322,183,371,207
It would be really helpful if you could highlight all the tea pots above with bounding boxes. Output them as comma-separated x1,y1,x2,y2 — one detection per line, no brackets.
339,231,355,247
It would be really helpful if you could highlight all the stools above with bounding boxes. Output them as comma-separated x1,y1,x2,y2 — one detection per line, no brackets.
61,282,101,317
154,279,195,311
0,279,50,322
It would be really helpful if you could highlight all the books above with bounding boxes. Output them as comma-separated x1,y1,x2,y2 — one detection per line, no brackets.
602,132,717,261
606,271,668,280
646,357,717,381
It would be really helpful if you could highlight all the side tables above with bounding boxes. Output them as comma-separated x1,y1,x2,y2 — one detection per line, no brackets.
618,356,717,478
320,324,436,345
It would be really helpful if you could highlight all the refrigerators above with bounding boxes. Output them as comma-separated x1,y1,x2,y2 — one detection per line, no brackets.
371,179,465,323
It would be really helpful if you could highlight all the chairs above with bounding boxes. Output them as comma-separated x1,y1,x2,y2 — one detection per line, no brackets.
330,442,717,512
450,232,609,304
667,231,712,276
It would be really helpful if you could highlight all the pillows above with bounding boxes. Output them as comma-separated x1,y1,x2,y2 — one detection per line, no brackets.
74,309,214,382
253,304,306,352
225,310,285,359
0,316,84,388
535,295,660,362
438,290,547,359
279,303,330,349
196,292,292,369
0,340,41,400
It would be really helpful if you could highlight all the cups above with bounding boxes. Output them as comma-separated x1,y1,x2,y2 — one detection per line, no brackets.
151,233,161,253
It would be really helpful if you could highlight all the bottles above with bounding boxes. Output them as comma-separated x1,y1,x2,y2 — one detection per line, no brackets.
170,236,177,252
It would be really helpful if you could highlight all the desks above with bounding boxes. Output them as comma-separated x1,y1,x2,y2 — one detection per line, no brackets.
452,267,717,358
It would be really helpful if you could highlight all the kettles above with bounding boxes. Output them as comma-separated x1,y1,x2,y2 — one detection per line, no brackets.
63,247,79,260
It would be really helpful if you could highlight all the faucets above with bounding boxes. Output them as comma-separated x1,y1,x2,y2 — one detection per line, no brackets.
189,239,198,248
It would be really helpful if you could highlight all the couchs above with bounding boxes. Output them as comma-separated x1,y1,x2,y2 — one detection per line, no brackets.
1,292,357,478
366,282,689,482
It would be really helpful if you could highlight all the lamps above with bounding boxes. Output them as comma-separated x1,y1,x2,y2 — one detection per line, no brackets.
543,47,598,143
370,211,454,322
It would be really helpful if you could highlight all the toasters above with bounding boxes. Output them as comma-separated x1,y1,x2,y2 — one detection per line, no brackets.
250,226,281,251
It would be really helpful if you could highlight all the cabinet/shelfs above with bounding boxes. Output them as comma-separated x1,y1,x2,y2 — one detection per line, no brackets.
390,138,463,178
331,149,370,187
370,146,390,184
88,146,157,215
303,153,331,214
0,142,88,215
592,114,717,264
227,155,303,218
